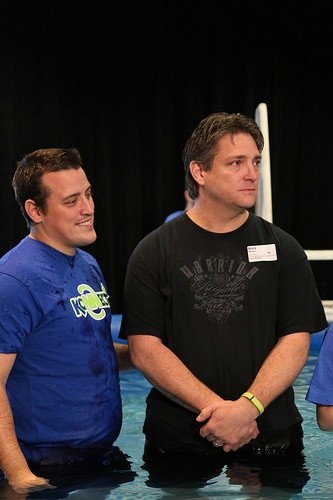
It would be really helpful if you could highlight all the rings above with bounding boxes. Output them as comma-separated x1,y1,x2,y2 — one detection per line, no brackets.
212,440,224,449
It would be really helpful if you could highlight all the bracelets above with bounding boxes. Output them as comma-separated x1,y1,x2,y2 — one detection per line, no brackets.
242,391,265,416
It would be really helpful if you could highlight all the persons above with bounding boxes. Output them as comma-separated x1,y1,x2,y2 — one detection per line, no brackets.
0,148,133,488
164,190,193,223
305,323,333,431
120,113,326,459
